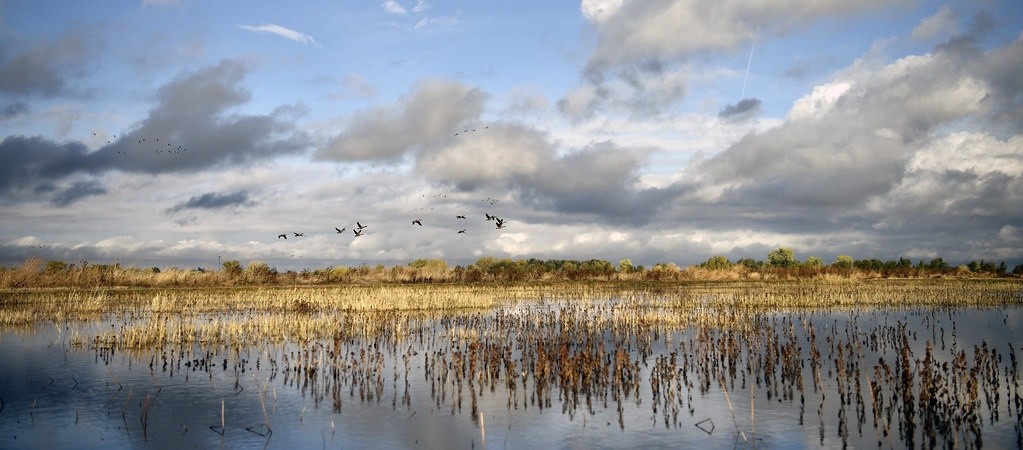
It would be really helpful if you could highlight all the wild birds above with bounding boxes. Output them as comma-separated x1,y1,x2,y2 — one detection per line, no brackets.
293,232,304,237
454,127,489,137
278,233,287,239
411,193,507,235
93,132,187,155
335,222,367,237
